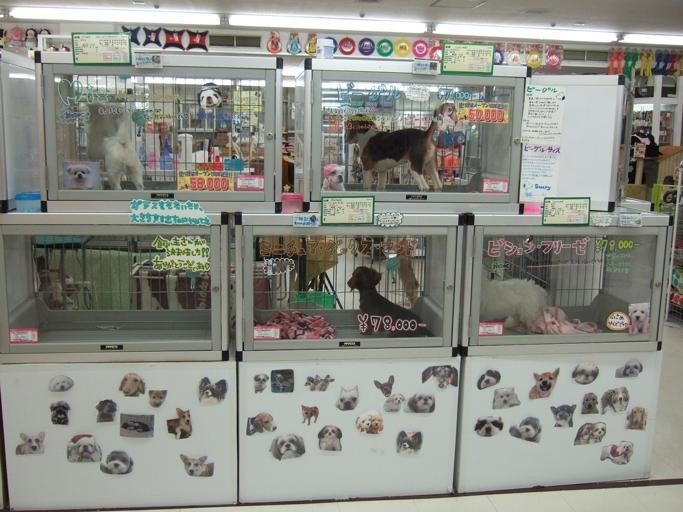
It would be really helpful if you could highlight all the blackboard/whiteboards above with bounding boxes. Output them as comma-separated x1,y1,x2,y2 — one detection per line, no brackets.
518,74,625,213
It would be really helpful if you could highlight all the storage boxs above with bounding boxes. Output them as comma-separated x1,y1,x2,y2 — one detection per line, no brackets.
230,212,459,502
300,55,523,216
33,49,284,215
453,207,658,499
1,207,238,511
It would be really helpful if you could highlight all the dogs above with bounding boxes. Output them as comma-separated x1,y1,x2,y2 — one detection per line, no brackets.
434,103,458,132
475,358,648,446
16,431,46,454
49,373,226,476
348,268,433,337
344,110,442,191
198,88,221,110
480,264,548,329
629,302,648,333
396,236,419,308
322,163,345,191
600,441,633,465
67,167,90,189
85,101,145,190
247,365,458,462
